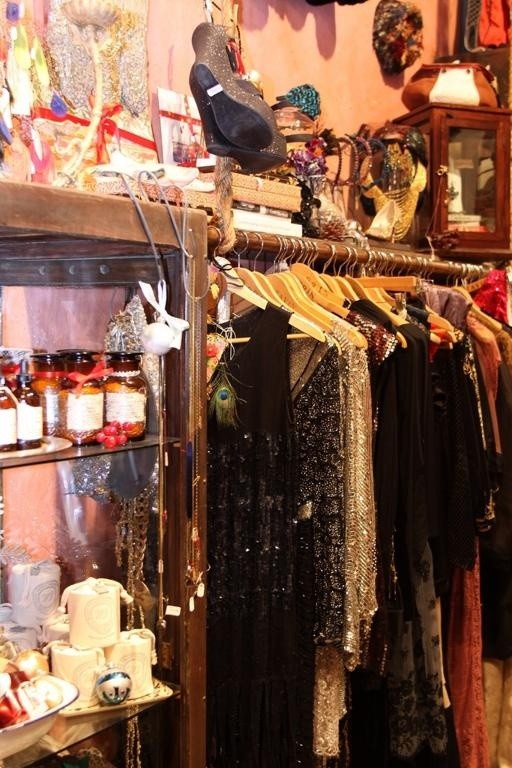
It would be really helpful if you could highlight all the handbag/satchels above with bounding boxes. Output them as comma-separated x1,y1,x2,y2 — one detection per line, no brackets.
402,63,499,111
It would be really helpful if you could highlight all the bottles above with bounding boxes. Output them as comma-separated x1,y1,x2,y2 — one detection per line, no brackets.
0,348,149,452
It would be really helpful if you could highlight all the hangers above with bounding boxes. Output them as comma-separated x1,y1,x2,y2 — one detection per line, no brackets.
208,225,503,349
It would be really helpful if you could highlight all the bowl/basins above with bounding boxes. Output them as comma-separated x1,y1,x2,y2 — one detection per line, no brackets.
0,673,83,761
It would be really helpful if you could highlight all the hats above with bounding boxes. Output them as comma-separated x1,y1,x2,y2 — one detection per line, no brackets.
373,125,427,167
372,0,424,74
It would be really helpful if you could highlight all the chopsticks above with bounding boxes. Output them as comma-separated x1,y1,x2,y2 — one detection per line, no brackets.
0,433,73,460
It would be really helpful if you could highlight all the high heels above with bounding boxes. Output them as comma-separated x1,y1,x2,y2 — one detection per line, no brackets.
190,23,287,174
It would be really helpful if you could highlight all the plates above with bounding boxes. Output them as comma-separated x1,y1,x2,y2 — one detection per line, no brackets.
58,675,174,719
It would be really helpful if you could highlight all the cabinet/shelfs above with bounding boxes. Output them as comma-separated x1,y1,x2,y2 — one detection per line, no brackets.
392,103,512,248
0,179,208,768
